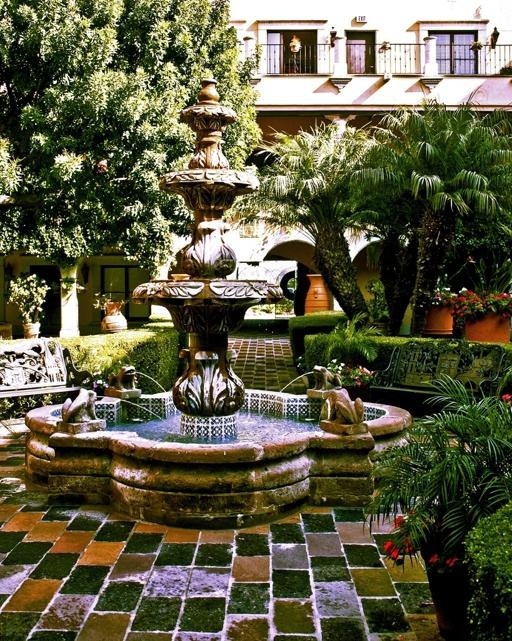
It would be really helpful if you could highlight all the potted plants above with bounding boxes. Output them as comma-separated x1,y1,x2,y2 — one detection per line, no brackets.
91,290,130,334
5,273,49,341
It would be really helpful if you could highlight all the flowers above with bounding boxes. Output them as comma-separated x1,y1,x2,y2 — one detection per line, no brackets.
418,281,511,319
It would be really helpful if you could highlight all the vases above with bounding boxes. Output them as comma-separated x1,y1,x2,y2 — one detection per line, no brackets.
422,305,511,343
